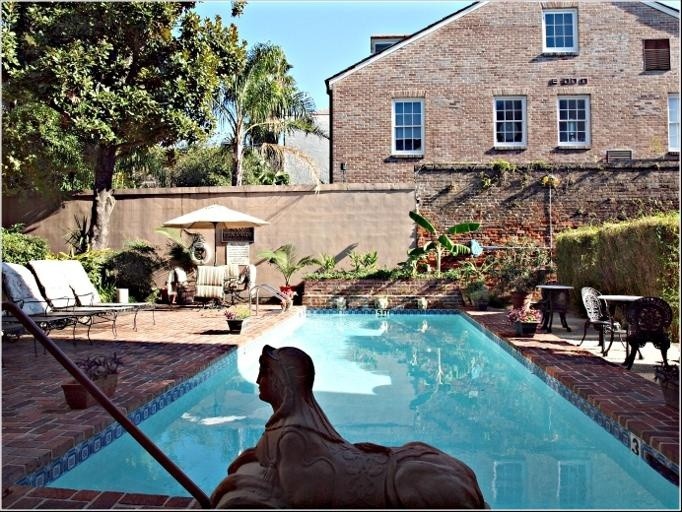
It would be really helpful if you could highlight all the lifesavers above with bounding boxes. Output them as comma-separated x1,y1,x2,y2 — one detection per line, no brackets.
189,243,210,265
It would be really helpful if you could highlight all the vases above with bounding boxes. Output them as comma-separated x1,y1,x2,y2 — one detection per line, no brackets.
226,319,244,333
515,323,537,338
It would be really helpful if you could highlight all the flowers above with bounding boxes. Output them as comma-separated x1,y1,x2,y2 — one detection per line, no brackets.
503,305,543,325
225,309,250,320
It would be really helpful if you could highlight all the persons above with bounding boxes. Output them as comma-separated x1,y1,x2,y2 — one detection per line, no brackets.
211,345,485,509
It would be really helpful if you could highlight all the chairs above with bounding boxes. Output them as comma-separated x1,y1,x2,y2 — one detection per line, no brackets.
576,286,675,371
166,263,257,305
2,258,157,357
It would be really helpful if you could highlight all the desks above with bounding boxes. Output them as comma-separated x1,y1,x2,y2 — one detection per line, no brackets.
533,280,576,333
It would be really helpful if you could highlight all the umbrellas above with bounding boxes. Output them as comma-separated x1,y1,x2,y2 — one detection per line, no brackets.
162,202,269,266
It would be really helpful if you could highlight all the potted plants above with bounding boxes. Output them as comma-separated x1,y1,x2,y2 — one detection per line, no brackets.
60,352,125,411
652,359,681,408
255,243,323,306
470,289,494,311
